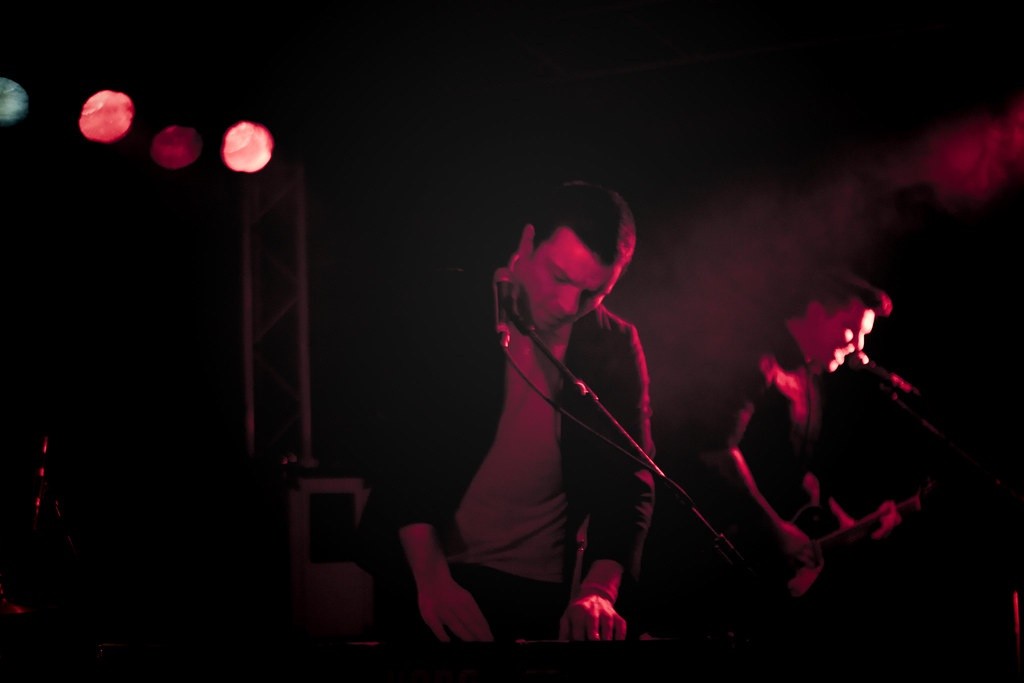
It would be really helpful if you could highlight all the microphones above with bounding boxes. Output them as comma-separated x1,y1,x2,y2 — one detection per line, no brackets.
493,265,516,348
848,351,918,396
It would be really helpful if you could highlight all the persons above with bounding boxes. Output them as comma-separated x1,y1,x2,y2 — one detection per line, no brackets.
674,271,894,625
352,180,656,643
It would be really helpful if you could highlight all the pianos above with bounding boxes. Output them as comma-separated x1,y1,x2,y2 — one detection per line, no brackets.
308,627,759,683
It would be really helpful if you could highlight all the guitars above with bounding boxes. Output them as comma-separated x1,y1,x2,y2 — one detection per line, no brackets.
772,477,941,598
